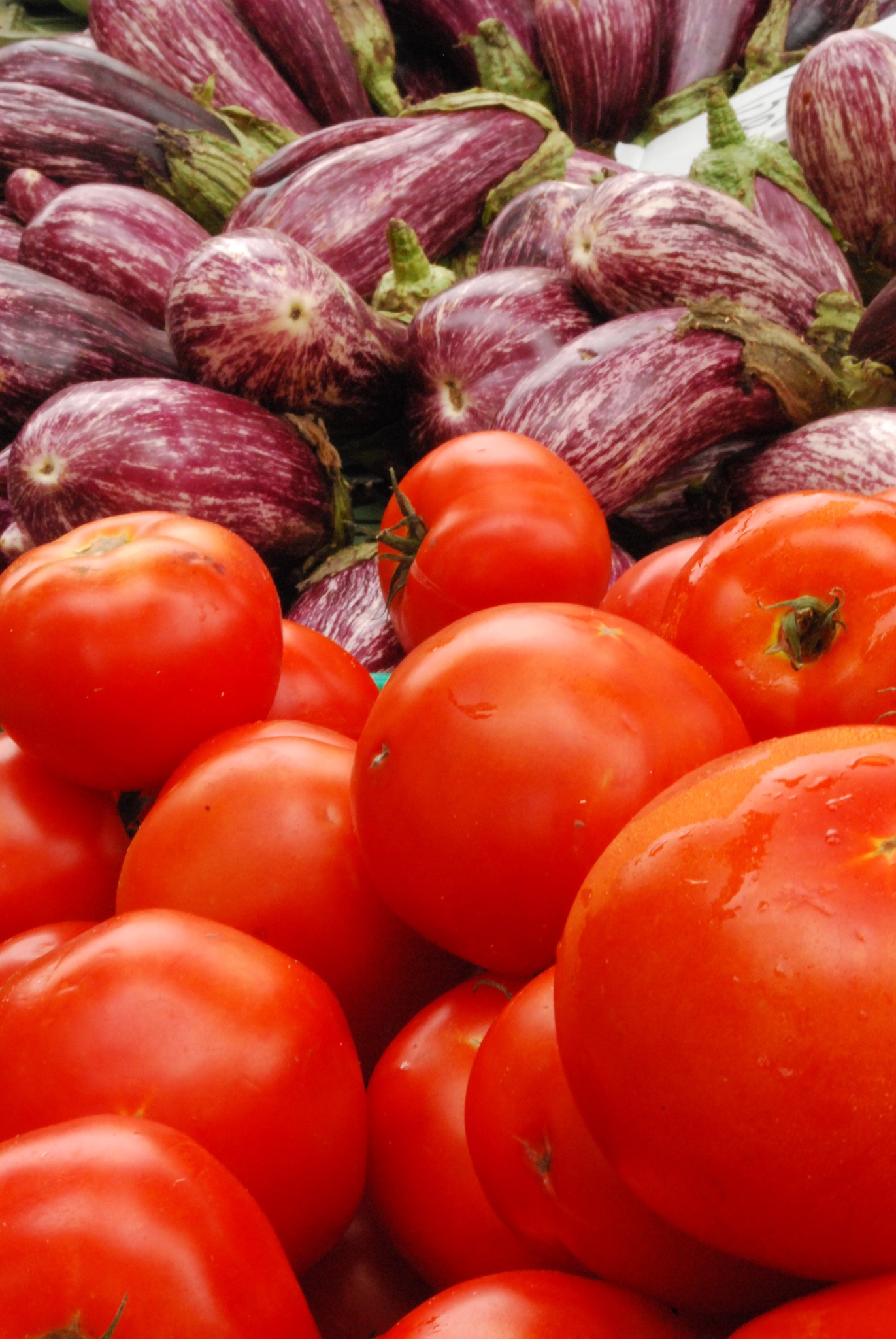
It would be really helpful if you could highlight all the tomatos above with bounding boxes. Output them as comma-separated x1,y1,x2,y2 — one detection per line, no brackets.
0,431,896,1338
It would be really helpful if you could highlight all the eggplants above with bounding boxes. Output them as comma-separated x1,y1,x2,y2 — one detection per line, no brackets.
0,1,896,673
